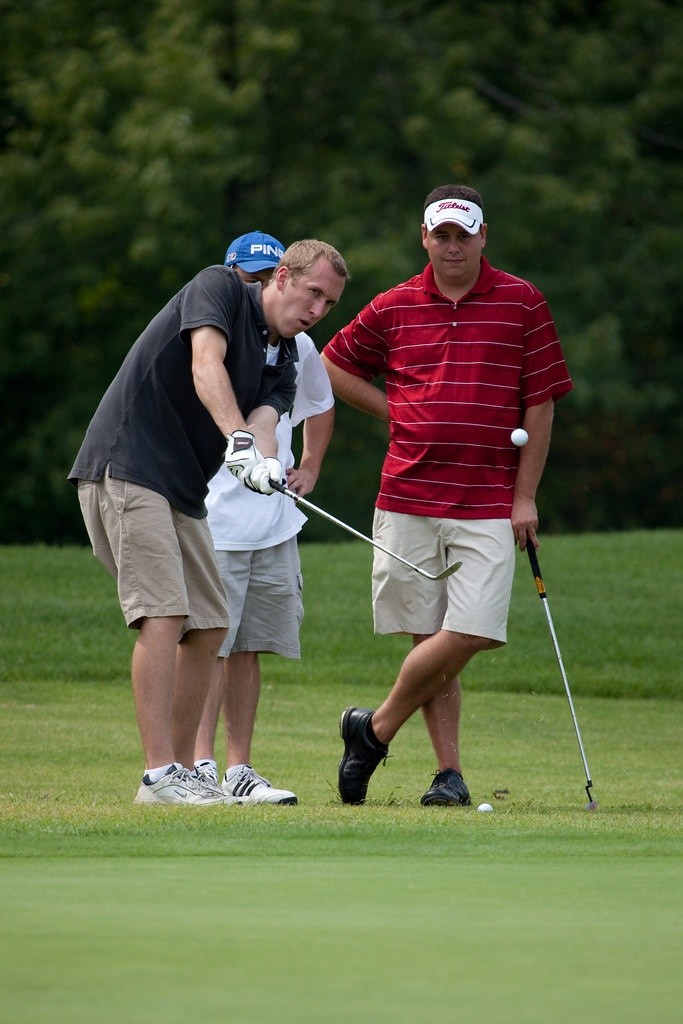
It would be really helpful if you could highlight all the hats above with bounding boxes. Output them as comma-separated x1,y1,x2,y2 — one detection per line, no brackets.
424,197,484,235
224,230,285,272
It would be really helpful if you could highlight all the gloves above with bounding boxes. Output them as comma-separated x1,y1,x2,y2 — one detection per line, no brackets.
224,430,264,478
239,457,287,496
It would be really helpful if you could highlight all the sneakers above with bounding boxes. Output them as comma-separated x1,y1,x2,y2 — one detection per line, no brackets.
420,768,472,807
131,763,241,811
338,706,391,805
193,762,219,789
222,763,298,807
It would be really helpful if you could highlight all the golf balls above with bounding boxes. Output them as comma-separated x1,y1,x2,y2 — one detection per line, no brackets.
477,804,492,812
510,425,529,446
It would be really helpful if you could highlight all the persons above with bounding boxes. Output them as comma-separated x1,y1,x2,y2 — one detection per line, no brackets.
74,239,348,809
321,181,556,806
195,230,334,805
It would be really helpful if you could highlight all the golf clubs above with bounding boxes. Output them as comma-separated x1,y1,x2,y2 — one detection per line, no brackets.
268,480,464,581
525,540,601,813
